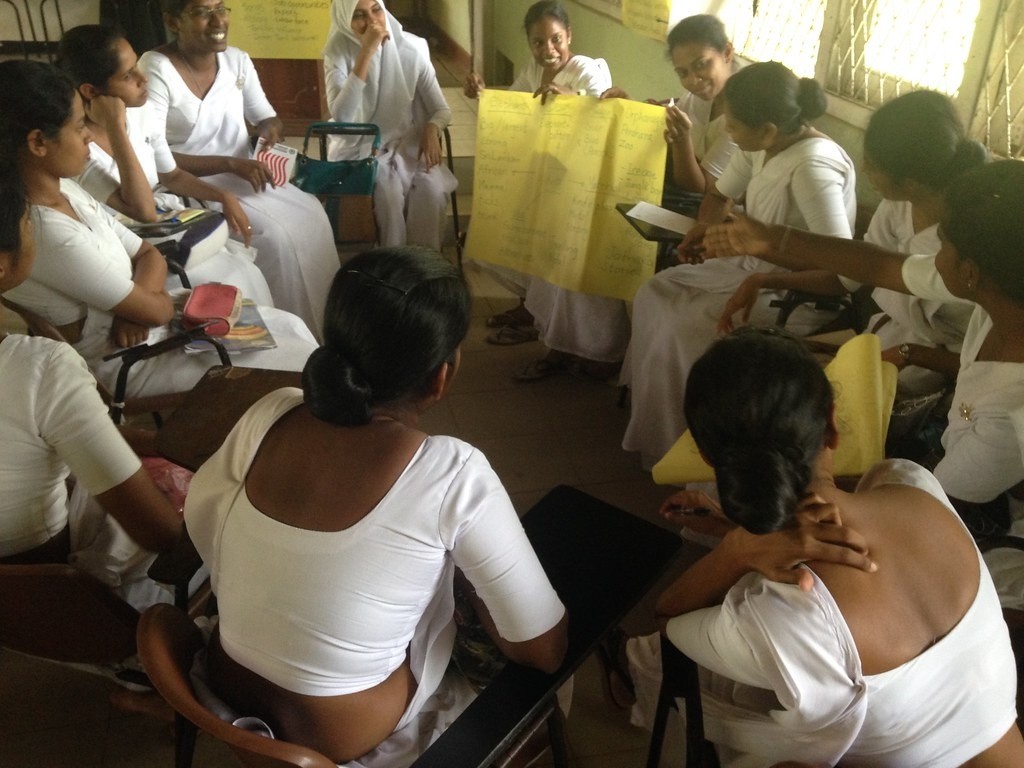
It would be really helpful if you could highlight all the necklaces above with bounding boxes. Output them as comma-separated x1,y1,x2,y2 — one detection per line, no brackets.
178,50,198,83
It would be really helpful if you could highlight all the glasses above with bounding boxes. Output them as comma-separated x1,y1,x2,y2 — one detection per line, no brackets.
179,4,231,20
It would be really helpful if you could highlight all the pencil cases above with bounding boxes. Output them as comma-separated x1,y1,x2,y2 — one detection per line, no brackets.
180,282,243,337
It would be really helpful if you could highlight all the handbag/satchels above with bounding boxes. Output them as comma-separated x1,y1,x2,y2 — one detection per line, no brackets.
181,282,241,338
294,122,381,244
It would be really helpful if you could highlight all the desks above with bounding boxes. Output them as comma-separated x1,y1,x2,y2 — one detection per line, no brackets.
152,363,303,471
129,207,219,236
405,482,685,768
616,204,696,244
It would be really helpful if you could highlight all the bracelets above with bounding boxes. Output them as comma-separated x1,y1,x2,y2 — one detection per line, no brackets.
779,225,791,252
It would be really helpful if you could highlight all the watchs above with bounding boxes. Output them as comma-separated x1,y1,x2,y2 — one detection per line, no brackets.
899,343,912,364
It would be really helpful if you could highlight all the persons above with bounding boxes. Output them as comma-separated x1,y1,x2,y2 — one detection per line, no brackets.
614,323,1024,768
323,0,457,251
616,62,1024,552
0,0,342,603
459,0,746,381
180,241,574,768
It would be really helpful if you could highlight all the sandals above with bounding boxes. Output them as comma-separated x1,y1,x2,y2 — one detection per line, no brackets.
599,629,635,710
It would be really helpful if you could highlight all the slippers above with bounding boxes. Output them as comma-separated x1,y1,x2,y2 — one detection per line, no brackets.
514,356,566,380
486,309,533,326
487,324,539,344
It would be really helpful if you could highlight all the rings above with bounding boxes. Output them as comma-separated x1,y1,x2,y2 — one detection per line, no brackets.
248,225,252,230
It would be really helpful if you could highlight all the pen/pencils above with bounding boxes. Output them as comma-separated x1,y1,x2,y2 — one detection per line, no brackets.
665,508,712,516
690,247,706,257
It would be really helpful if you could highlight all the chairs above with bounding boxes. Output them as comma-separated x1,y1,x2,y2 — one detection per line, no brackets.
0,122,1024,768
